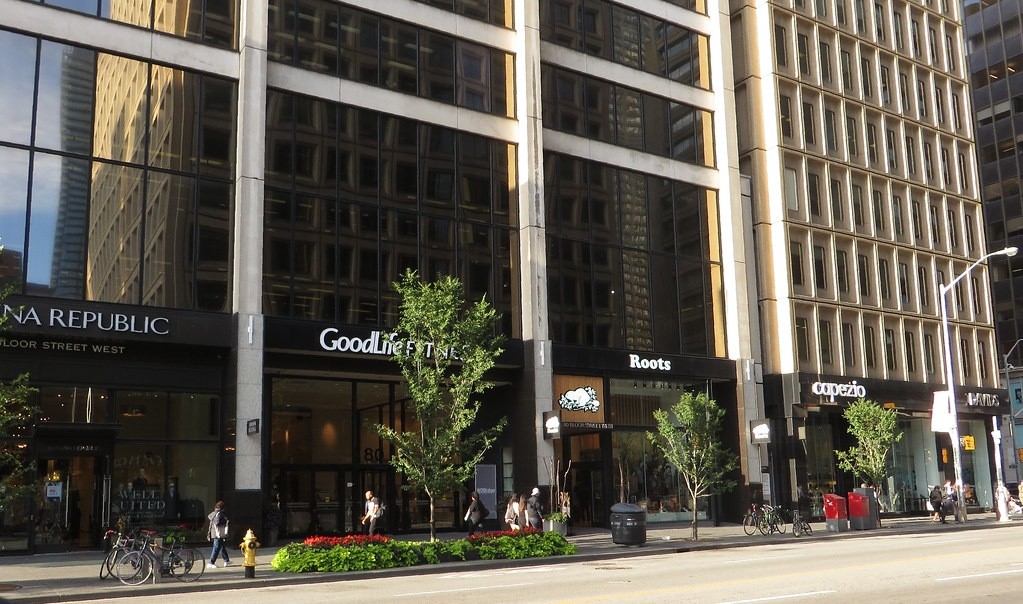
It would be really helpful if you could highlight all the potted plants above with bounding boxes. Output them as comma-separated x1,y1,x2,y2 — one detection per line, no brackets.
542,512,572,536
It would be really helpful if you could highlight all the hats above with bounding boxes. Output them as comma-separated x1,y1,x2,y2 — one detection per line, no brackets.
531,487,540,495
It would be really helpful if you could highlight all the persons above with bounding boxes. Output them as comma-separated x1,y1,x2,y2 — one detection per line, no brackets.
464,492,487,536
1018,480,1023,506
929,485,943,523
942,479,958,522
161,482,181,521
861,483,885,528
518,493,532,528
207,500,234,568
132,467,148,522
115,482,132,513
506,491,520,530
951,485,958,523
361,491,382,536
995,482,1011,512
528,488,544,528
67,481,82,515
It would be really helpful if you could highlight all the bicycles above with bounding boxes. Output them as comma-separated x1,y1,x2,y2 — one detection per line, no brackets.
786,508,813,537
743,503,789,536
99,528,206,586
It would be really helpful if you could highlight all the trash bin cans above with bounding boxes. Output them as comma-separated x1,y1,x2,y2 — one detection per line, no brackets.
610,503,647,545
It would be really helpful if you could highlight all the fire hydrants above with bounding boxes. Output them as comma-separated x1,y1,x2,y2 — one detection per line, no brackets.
238,528,260,579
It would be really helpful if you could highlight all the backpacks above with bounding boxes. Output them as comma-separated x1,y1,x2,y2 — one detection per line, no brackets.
505,504,519,525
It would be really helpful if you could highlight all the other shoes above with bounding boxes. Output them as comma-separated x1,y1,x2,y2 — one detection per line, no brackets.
224,560,233,567
207,563,218,568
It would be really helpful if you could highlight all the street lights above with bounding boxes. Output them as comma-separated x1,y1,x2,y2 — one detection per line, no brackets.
938,244,1019,525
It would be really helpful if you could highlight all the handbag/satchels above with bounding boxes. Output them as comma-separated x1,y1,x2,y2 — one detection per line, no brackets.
215,512,226,526
372,507,383,518
472,510,483,526
483,507,490,518
